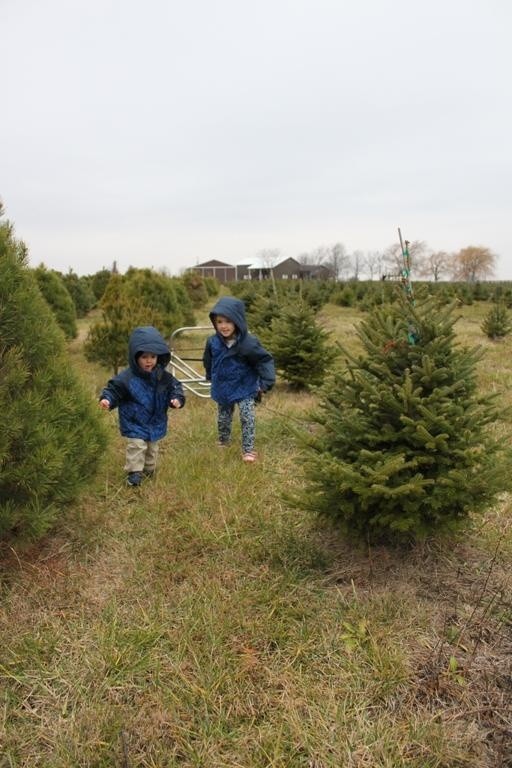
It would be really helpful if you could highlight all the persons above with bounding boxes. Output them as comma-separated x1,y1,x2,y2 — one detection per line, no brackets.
99,326,185,487
202,294,276,462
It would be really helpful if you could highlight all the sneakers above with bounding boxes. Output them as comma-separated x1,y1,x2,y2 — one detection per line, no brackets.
242,450,258,463
127,471,143,486
